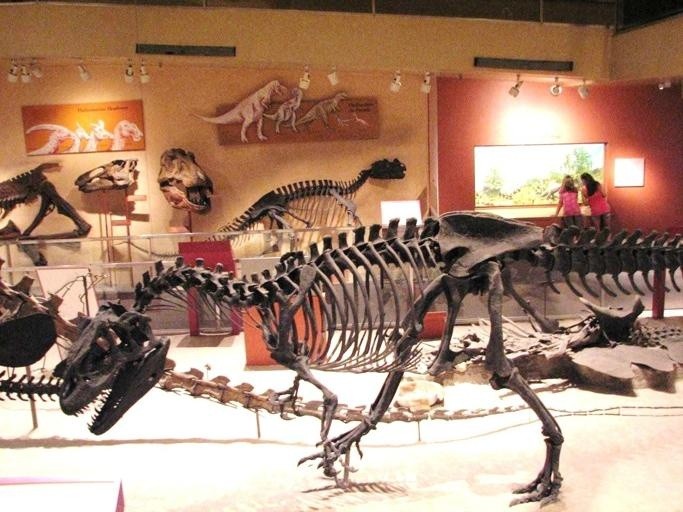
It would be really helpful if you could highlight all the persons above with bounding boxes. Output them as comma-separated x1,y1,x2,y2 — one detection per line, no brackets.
579,172,612,231
551,174,583,225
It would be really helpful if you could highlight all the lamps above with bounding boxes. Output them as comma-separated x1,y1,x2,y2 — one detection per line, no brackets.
124,64,150,84
389,69,431,93
550,77,589,99
299,64,338,89
507,73,523,96
76,64,90,82
6,58,41,84
